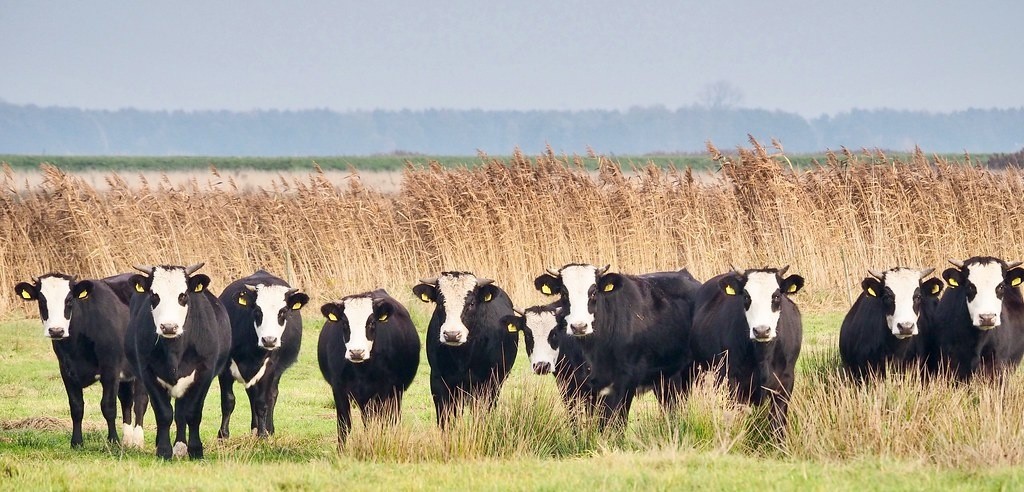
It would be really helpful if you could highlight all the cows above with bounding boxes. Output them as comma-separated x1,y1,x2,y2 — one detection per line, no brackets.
413,270,519,430
14,273,147,449
499,263,805,447
839,256,1024,386
218,270,309,440
317,289,420,452
125,262,232,461
